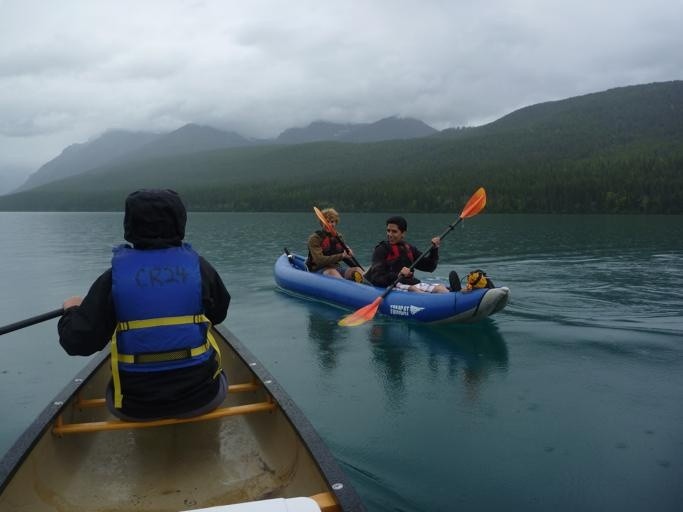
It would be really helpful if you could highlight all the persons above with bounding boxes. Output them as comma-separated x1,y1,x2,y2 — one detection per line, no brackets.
364,216,462,294
304,208,366,283
56,187,232,424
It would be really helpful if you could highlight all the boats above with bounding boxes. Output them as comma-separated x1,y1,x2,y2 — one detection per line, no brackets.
272,251,512,327
0,318,364,512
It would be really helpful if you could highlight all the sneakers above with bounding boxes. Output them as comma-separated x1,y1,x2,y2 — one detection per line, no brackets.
449,271,461,292
353,271,362,283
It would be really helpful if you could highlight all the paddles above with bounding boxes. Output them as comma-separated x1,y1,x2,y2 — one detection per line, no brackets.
313,207,366,271
337,187,487,327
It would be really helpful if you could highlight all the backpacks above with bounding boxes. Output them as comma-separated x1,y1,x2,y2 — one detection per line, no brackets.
461,269,495,290
